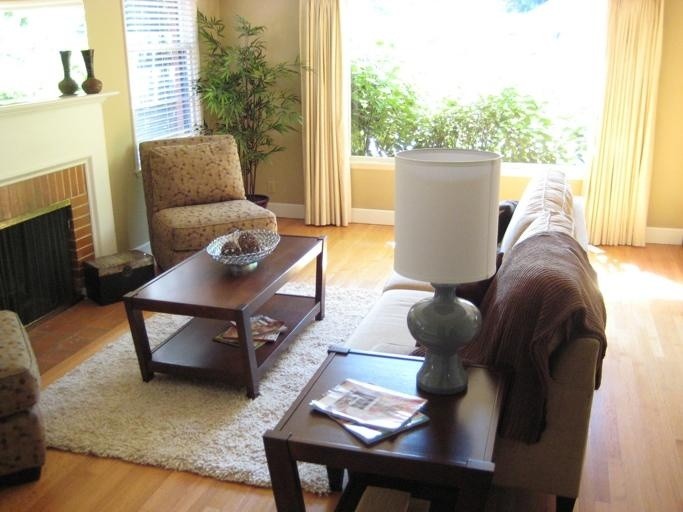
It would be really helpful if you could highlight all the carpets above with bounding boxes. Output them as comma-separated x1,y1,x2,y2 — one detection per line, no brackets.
30,280,383,494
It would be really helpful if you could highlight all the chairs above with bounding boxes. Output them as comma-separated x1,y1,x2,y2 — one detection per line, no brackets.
138,135,278,274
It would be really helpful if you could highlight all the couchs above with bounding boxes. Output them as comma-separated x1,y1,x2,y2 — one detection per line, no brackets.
347,170,604,512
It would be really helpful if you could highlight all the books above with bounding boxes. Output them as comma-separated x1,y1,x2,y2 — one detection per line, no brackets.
307,376,430,433
328,409,431,447
212,313,287,354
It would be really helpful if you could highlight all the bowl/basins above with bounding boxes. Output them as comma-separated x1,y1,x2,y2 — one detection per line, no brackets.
206,229,280,265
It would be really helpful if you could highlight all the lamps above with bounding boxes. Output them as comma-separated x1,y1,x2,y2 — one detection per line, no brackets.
390,144,503,399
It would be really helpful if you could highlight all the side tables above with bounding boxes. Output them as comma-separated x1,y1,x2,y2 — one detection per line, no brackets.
259,346,510,512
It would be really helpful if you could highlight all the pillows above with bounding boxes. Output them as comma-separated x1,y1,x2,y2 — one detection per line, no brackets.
148,137,246,208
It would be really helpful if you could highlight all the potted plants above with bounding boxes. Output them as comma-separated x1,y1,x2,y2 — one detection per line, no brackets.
190,6,314,209
